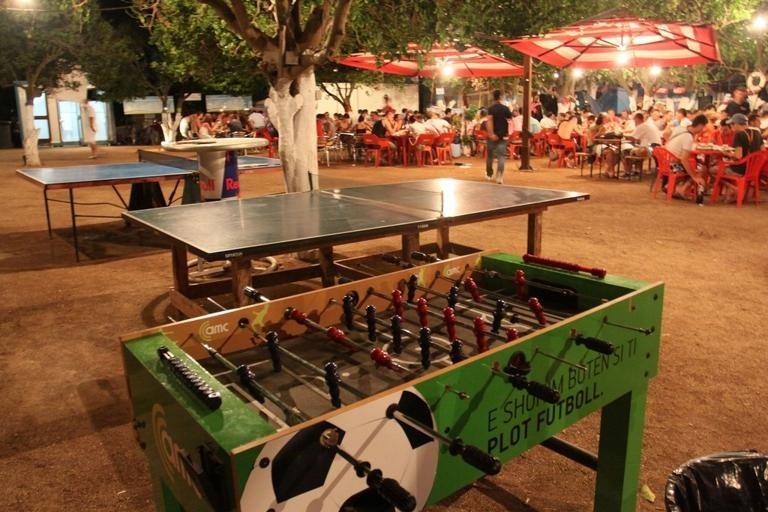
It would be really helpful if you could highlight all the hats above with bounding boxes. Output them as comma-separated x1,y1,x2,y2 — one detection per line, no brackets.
427,105,442,114
725,113,749,125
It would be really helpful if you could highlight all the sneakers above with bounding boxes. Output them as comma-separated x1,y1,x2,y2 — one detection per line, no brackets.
496,176,503,184
485,171,494,180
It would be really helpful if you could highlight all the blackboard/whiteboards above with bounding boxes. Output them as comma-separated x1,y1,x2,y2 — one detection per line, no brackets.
665,451,768,512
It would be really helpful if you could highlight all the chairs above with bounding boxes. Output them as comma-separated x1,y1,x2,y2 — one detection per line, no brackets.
262,128,768,208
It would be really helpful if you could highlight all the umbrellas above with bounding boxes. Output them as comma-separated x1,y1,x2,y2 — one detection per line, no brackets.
327,32,525,78
498,16,727,71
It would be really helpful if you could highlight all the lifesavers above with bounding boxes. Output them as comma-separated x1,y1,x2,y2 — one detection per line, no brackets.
747,72,766,92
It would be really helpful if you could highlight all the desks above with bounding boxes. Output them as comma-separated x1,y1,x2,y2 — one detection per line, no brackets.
122,179,590,315
15,149,282,262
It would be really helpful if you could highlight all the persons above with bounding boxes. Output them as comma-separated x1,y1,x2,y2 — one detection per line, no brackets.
485,90,513,183
509,86,767,208
179,94,488,165
81,99,99,160
